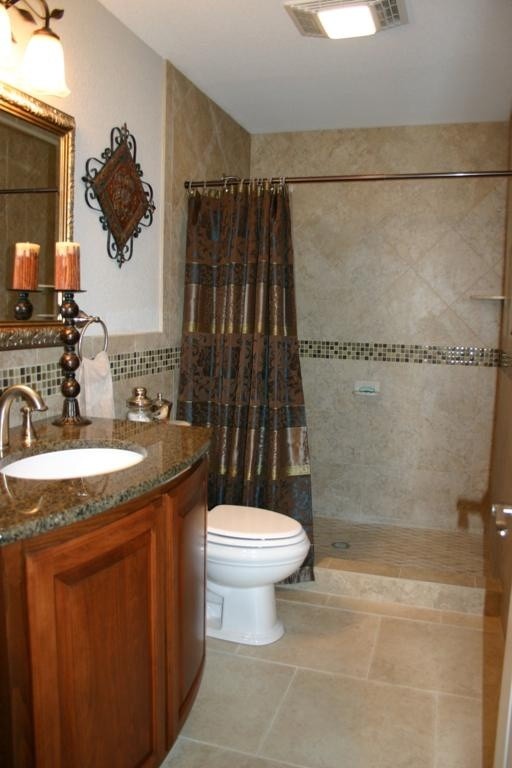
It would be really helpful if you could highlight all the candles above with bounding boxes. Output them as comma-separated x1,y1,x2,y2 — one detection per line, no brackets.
51,237,86,295
8,237,41,290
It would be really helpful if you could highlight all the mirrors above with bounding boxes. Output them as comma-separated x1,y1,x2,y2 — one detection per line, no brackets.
0,76,102,356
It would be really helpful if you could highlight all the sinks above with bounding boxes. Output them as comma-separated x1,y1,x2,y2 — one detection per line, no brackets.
0,438,149,480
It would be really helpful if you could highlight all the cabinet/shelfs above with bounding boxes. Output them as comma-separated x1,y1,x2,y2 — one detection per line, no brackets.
1,413,224,766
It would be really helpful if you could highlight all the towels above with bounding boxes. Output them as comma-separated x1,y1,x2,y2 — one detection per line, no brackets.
73,348,119,425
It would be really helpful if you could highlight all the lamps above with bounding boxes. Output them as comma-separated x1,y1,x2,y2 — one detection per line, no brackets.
281,1,419,45
0,1,82,102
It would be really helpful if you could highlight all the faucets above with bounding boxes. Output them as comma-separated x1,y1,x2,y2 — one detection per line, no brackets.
0,384,49,450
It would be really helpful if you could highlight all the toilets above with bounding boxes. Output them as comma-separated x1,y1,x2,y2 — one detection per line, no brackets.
164,418,312,647
